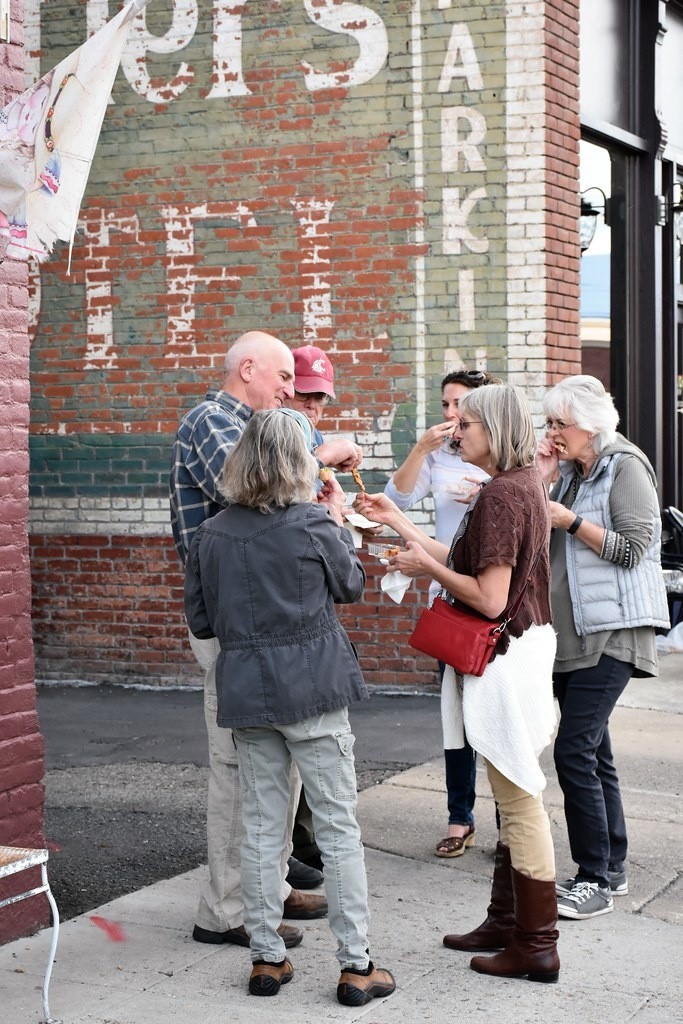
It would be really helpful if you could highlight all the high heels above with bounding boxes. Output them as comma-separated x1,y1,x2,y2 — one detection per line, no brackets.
434,824,477,857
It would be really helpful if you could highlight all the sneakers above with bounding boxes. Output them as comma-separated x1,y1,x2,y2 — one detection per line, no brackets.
249,956,294,996
337,961,396,1006
556,882,614,919
554,871,629,897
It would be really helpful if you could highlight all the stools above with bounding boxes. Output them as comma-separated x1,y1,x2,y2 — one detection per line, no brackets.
0,845,60,1024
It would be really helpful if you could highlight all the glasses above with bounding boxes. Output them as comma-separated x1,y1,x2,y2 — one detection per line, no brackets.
542,423,576,431
467,369,485,389
459,419,483,430
295,391,331,405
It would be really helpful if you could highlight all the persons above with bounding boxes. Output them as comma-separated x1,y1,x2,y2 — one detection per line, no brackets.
352,385,558,983
169,331,363,950
535,375,670,919
183,410,396,1006
384,371,516,857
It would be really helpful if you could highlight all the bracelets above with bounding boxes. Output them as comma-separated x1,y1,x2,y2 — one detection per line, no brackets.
566,516,582,535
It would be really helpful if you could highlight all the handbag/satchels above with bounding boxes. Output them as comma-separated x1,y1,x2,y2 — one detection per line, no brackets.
408,596,500,676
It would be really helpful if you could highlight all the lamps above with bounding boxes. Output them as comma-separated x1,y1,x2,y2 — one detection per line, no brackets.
580,198,600,258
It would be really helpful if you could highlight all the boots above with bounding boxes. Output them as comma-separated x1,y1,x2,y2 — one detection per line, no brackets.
443,842,518,953
470,865,560,983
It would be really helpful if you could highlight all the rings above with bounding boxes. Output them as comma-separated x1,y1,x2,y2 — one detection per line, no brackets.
443,437,449,441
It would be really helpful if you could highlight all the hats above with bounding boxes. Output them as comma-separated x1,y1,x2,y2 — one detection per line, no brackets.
291,345,336,399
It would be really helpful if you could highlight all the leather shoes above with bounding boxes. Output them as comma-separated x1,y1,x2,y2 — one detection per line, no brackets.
293,828,324,873
282,888,328,920
192,922,303,949
287,856,325,890
